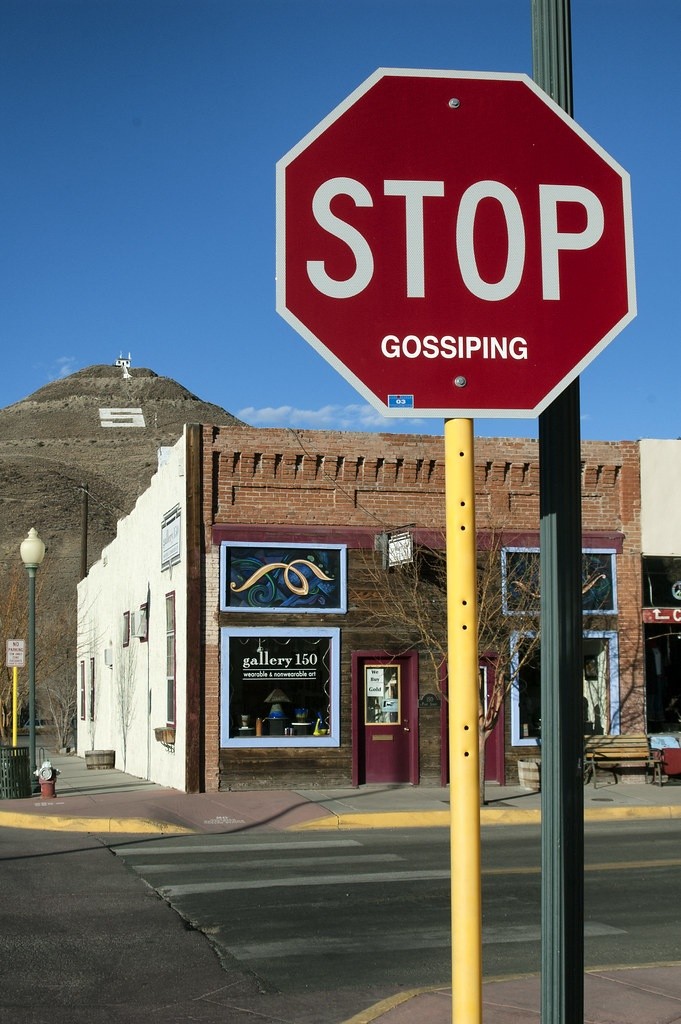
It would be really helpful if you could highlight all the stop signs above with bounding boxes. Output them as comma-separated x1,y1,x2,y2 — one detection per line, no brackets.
275,68,638,417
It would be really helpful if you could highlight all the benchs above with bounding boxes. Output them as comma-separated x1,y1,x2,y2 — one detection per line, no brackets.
583,733,664,788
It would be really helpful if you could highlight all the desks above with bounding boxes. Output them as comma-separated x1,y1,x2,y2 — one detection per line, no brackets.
265,717,288,735
238,728,255,735
292,722,311,735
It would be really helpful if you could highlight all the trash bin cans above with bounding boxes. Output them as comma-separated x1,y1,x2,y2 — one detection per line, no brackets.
0,746,32,799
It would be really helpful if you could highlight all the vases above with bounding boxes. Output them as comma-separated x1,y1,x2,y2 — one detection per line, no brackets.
295,708,308,722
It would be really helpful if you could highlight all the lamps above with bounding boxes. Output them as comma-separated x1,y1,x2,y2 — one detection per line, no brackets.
264,688,293,717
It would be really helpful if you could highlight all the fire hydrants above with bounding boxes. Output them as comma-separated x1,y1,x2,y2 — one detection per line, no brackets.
33,761,62,799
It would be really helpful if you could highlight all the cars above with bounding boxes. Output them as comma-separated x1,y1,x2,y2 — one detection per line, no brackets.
24,718,53,734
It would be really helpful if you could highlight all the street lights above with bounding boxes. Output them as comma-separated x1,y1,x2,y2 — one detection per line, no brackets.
19,527,46,792
